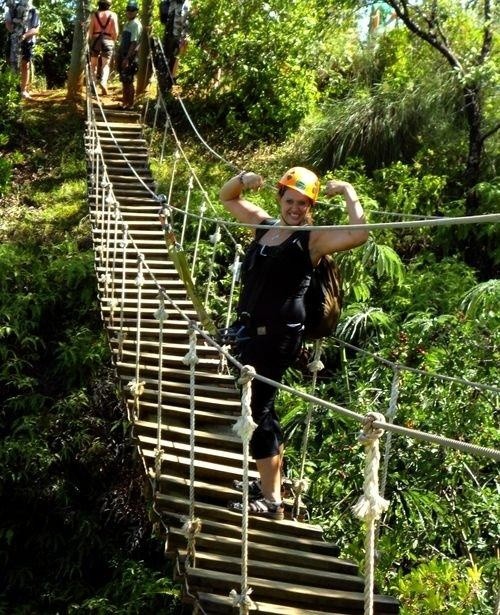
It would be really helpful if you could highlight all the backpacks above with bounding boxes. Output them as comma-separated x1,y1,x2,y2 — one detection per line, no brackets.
241,219,345,339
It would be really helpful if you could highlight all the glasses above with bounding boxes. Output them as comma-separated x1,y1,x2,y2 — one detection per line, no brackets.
283,193,309,205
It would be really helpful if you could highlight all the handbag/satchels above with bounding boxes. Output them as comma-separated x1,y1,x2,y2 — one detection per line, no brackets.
240,315,274,352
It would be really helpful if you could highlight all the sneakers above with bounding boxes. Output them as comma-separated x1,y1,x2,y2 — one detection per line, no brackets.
227,479,285,520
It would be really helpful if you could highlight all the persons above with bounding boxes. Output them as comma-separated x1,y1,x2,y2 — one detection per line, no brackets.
5,0,41,98
116,0,142,110
87,0,118,95
219,167,369,526
160,0,190,76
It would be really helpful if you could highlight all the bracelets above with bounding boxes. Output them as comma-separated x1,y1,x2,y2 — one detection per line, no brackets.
239,170,248,184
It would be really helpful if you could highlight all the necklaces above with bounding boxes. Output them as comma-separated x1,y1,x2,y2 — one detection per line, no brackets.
272,231,285,238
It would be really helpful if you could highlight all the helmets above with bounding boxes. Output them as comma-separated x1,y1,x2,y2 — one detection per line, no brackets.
278,167,319,202
